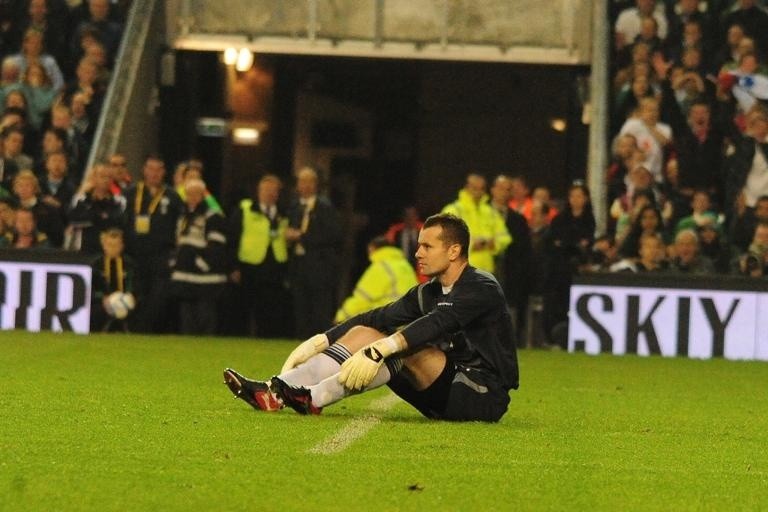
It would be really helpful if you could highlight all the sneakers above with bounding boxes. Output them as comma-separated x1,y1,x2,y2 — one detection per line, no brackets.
224,368,284,411
271,374,322,415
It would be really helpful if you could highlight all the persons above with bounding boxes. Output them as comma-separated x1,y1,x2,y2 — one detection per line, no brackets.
223,213,520,422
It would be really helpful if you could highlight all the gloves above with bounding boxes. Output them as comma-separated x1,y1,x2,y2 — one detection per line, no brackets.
282,334,329,374
338,331,408,391
